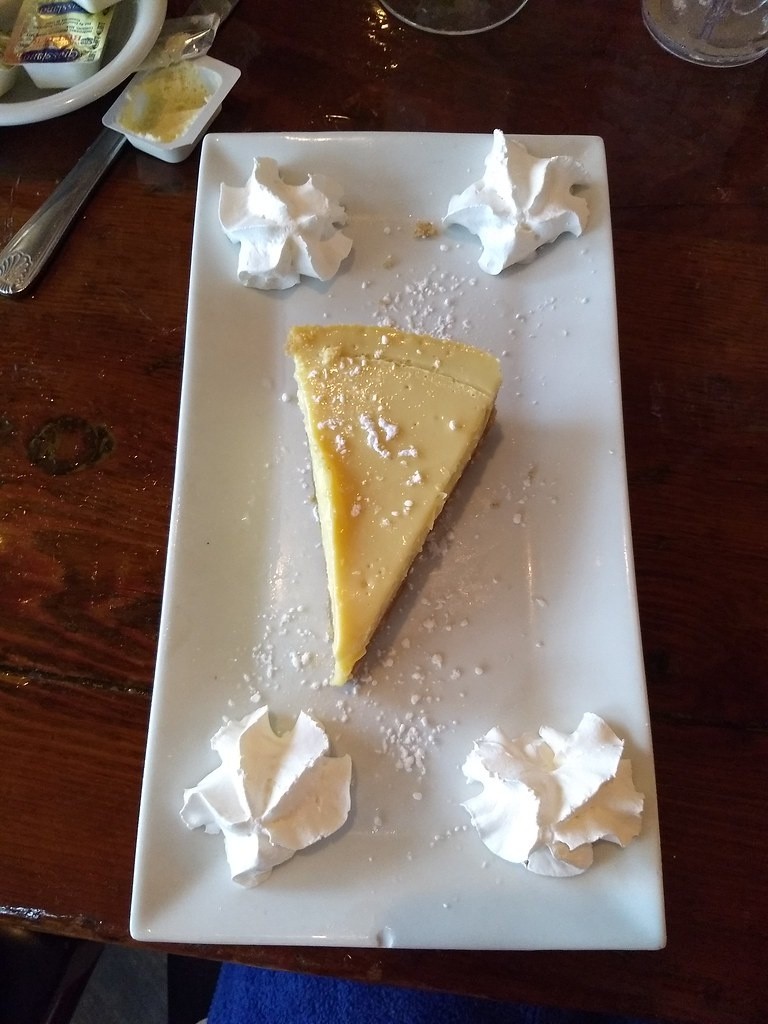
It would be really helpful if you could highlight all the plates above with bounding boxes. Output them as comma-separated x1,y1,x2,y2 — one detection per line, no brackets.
127,130,668,953
378,0,528,36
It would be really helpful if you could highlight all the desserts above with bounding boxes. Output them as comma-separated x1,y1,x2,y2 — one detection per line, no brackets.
287,324,505,684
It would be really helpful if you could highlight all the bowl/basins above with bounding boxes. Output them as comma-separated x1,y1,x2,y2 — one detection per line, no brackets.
0,0,168,127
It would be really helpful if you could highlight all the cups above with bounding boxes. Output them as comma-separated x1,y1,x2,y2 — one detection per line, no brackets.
640,0,768,68
